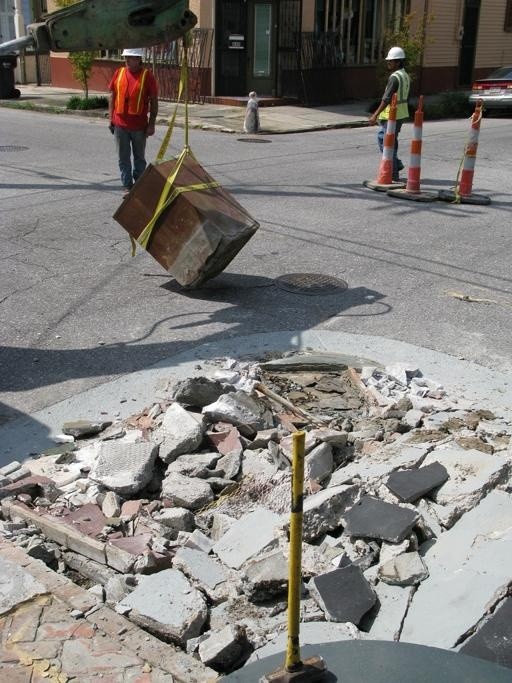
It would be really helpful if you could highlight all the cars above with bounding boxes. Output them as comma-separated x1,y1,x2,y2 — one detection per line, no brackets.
466,64,511,115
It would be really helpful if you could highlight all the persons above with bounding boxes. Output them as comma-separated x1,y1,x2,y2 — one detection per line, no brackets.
108,48,159,199
368,47,414,181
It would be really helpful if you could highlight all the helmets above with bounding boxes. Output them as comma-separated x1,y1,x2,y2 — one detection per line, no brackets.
385,46,406,61
121,48,144,57
